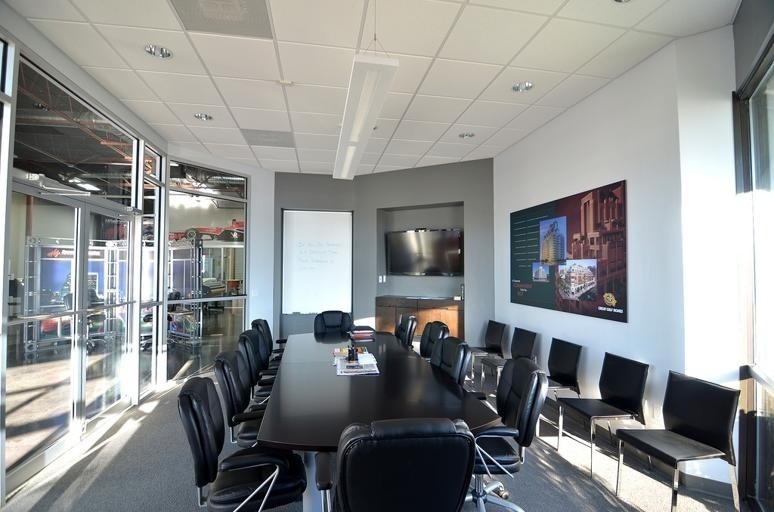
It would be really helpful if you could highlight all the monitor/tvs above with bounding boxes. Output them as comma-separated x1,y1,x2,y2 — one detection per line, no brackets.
384,228,464,277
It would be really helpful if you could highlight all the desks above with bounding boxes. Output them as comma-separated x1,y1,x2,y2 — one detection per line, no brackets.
256,331,502,453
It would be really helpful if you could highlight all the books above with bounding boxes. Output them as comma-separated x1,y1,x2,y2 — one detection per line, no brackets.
348,326,377,343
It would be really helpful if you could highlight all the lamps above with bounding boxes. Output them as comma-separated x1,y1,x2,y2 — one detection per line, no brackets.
332,0,399,181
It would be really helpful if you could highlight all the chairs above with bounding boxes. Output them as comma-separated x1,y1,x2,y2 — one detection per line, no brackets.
480,327,537,388
535,338,596,437
430,336,472,385
251,319,287,367
616,369,741,512
464,356,549,512
315,417,475,512
65,288,181,355
467,320,506,380
213,351,267,448
557,352,654,480
395,312,419,346
177,377,307,512
420,321,449,358
314,311,353,333
238,330,281,405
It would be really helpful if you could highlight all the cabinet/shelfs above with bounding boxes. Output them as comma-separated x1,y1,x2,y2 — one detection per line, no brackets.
418,297,463,339
375,295,418,336
